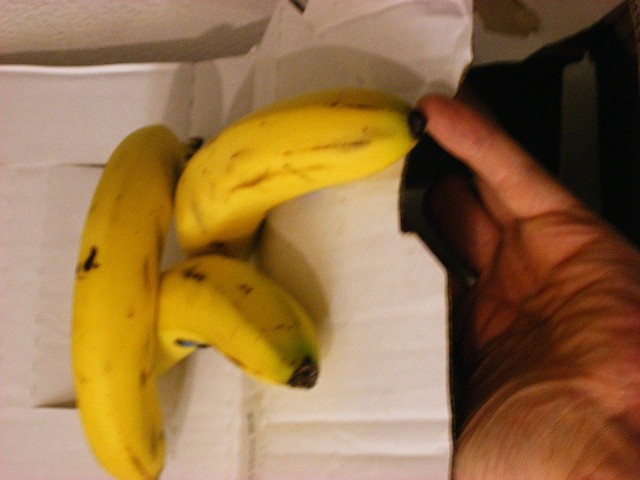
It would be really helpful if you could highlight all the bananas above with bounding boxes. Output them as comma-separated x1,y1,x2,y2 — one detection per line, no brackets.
71,125,203,476
158,254,320,389
174,89,427,260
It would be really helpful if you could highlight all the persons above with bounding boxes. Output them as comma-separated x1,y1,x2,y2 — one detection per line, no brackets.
415,91,640,480
